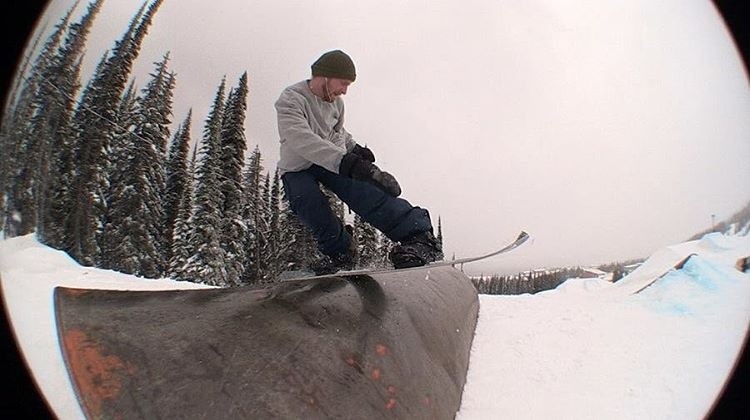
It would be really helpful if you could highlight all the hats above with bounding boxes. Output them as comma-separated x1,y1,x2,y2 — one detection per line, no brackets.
312,50,355,82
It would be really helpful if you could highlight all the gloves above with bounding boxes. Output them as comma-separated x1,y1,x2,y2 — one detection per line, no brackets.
350,145,375,163
356,159,402,197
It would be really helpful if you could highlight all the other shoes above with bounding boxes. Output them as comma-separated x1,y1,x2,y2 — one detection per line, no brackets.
389,231,446,268
311,245,359,276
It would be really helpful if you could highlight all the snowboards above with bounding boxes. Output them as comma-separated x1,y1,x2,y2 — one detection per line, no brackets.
281,232,530,282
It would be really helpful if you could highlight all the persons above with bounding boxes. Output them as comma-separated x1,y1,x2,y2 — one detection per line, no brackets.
275,51,444,276
